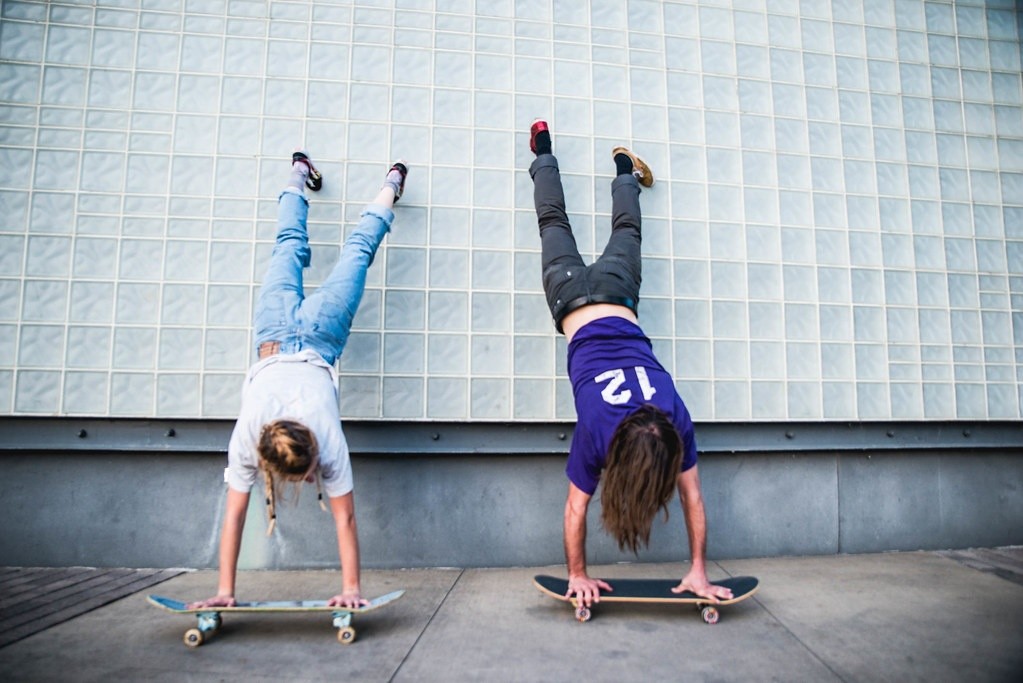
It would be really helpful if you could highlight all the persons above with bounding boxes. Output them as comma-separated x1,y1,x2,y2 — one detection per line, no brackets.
528,119,732,610
186,151,408,608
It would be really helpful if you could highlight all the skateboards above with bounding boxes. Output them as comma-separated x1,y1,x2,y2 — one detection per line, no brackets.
146,590,405,647
532,573,760,625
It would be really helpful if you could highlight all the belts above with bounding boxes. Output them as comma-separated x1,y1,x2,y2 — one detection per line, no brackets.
556,295,638,334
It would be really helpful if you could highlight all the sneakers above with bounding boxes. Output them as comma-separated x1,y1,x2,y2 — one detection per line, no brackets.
386,159,409,204
613,146,655,187
291,151,322,192
530,119,548,154
